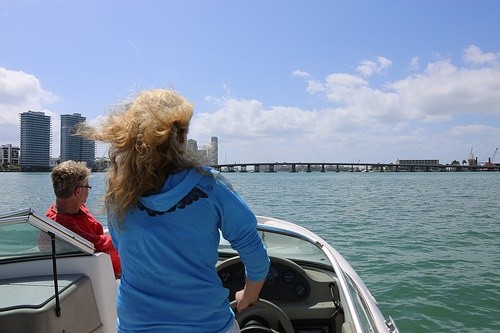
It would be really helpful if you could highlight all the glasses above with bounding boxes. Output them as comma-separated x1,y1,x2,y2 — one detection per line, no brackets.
76,185,92,190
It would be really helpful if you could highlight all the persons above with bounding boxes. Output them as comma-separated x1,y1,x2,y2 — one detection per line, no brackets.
43,159,123,276
78,88,271,333
